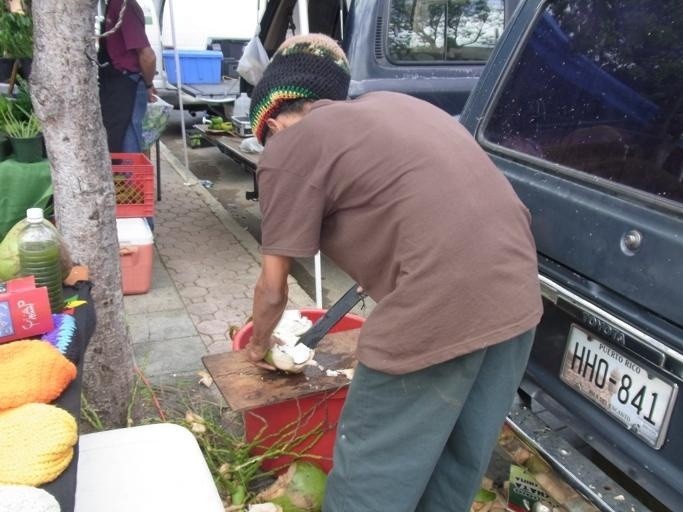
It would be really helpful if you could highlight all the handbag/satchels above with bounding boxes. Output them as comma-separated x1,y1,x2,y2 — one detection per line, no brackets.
140,93,174,148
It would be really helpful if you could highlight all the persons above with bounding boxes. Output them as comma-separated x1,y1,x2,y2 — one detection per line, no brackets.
243,33,543,512
96,1,156,230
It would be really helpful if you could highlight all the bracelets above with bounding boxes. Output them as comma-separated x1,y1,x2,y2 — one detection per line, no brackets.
145,83,153,89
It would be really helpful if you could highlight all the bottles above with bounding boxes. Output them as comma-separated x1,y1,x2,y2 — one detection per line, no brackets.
18,207,65,312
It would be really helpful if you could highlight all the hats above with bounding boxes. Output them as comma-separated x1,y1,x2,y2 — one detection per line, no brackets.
248,34,350,147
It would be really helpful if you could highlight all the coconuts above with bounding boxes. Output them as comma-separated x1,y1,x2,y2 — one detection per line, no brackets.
0,215,52,280
253,307,315,374
261,461,327,511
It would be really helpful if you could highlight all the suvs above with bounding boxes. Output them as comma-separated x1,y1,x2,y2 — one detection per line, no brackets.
191,0,530,199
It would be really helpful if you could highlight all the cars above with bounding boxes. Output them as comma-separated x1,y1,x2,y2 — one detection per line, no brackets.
398,0,682,506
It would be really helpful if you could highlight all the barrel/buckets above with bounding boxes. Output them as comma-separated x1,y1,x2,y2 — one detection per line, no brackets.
233,92,251,117
233,308,366,480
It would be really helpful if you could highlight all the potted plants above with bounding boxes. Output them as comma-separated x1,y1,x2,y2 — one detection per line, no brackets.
0,97,44,163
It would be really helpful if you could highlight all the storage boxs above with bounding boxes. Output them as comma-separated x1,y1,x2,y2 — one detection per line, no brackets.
112,215,154,296
162,49,223,85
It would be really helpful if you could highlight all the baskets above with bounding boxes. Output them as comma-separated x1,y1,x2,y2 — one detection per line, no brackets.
110,153,154,218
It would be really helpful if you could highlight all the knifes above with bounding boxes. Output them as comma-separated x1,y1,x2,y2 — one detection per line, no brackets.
295,284,368,352
